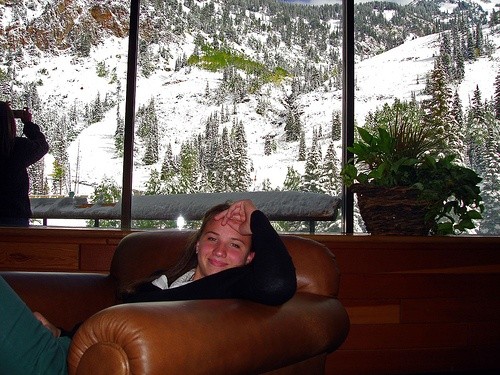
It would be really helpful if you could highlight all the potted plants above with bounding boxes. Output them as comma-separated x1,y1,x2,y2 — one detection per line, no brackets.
340,113,485,236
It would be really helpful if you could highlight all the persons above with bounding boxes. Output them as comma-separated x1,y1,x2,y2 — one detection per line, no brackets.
0,198,298,375
0,101,50,228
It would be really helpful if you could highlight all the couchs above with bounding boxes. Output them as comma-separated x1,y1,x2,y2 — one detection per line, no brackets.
0,229,351,375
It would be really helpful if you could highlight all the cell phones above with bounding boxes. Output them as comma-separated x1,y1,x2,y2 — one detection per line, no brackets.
9,110,26,118
